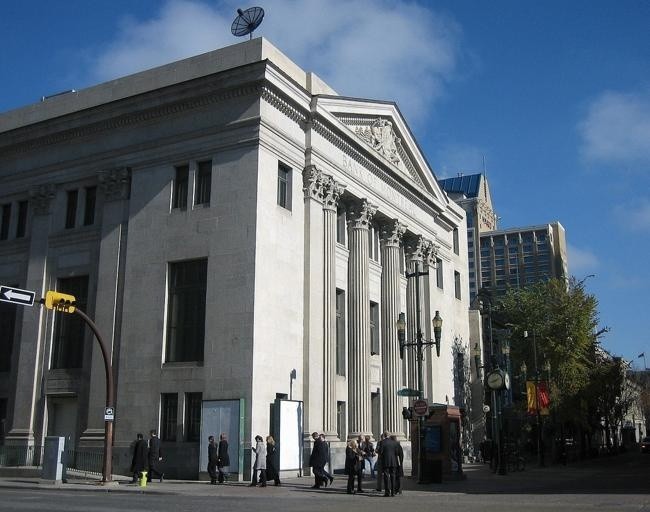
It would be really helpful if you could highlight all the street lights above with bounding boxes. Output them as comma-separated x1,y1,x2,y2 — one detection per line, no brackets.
474,292,516,456
590,319,644,449
521,311,551,456
396,238,440,475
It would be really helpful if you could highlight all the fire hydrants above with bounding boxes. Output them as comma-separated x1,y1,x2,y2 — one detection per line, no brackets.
139,472,148,486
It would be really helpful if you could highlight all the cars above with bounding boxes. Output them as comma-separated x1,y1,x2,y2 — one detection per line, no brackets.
639,437,650,453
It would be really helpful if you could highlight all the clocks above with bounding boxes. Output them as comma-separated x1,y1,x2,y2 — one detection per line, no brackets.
487,373,503,389
504,373,510,390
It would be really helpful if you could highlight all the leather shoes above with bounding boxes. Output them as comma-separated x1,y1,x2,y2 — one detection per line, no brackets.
312,476,333,488
347,486,401,496
248,480,280,487
129,480,137,484
160,472,164,483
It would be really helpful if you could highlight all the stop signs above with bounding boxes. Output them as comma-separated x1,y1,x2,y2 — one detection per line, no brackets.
412,400,429,417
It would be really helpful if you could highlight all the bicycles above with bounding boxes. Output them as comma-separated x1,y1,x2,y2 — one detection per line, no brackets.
507,451,525,472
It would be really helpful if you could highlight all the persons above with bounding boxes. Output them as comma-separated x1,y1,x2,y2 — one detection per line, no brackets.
216,432,230,483
345,431,404,496
147,430,164,482
250,436,267,487
129,434,148,484
319,433,334,486
461,438,485,464
207,436,218,484
259,436,281,485
309,431,329,488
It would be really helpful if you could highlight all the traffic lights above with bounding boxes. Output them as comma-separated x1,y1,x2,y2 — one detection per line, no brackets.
45,291,76,314
